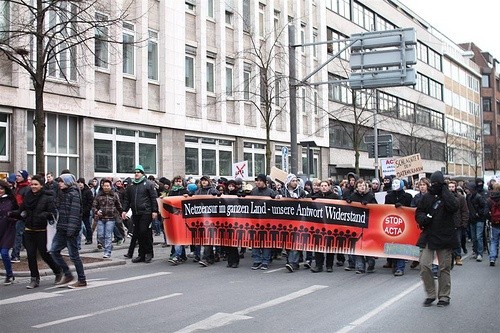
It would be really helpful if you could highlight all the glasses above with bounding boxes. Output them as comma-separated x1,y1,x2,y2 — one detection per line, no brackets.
218,182,226,185
291,180,297,183
133,171,142,174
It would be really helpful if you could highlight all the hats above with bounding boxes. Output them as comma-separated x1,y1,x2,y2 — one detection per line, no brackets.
7,173,16,183
59,174,77,187
347,172,360,182
218,177,228,183
430,171,444,184
79,178,85,184
18,170,28,181
134,165,144,173
284,174,297,185
187,184,198,193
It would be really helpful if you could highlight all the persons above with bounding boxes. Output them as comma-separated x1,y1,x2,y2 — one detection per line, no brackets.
0,165,500,289
415,171,458,307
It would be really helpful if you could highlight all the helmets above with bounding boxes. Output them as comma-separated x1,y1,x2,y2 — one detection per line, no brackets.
257,174,266,184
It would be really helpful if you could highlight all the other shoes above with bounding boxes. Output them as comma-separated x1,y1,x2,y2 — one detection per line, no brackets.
1,272,87,289
456,256,462,265
477,255,482,261
437,300,449,307
467,239,471,242
472,251,475,255
483,246,487,253
84,229,439,279
10,257,21,262
490,259,495,263
424,298,435,307
461,245,467,253
474,254,477,259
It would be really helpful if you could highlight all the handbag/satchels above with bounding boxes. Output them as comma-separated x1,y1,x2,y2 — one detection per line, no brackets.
45,209,60,252
